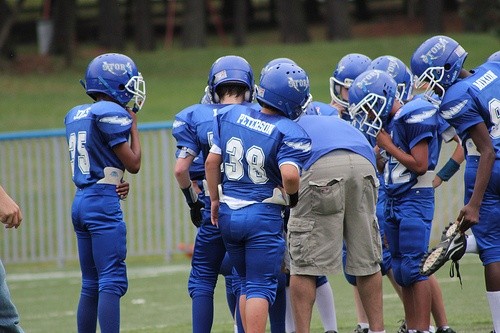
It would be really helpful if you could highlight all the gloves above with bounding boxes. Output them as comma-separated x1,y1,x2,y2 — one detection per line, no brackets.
179,183,209,227
287,192,299,208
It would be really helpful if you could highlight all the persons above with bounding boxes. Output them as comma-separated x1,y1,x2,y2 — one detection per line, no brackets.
286,115,388,333
172,35,500,333
346,69,443,333
204,62,311,333
64,52,142,333
0,183,25,333
410,35,500,333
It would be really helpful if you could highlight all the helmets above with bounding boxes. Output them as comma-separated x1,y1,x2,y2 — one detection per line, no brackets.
207,55,253,105
334,53,372,108
366,55,414,103
411,35,469,90
261,58,297,78
256,63,313,121
84,52,138,106
348,70,395,125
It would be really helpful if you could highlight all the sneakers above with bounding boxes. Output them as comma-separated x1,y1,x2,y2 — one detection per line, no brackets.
353,325,369,333
421,222,469,289
398,325,424,333
436,327,455,333
